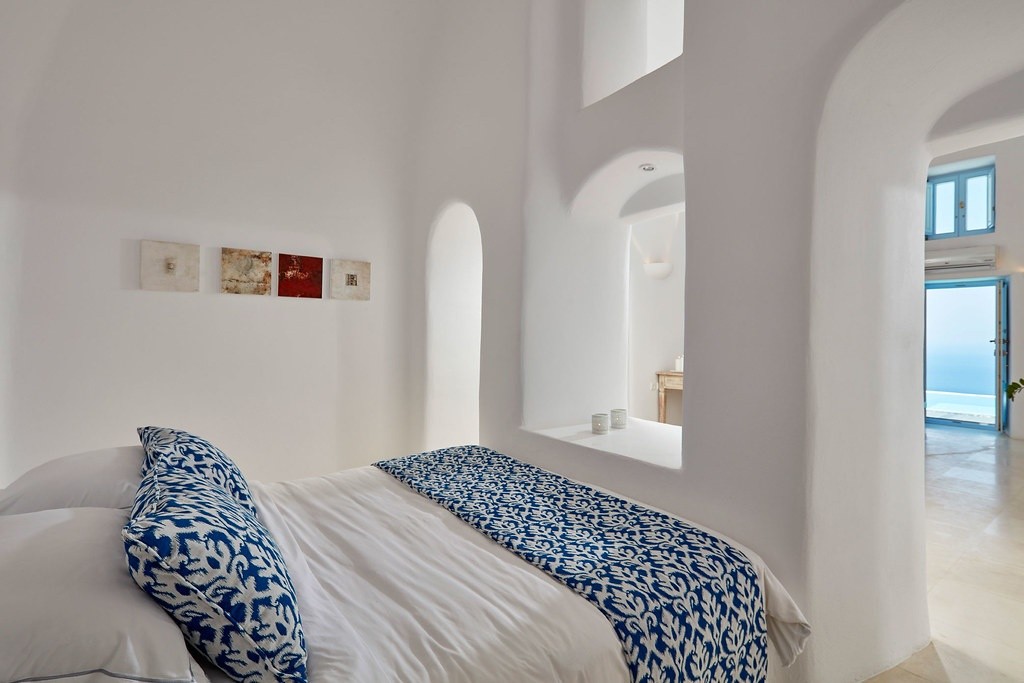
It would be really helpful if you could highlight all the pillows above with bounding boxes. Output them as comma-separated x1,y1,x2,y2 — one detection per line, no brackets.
0,507,211,683
138,426,258,520
0,446,146,516
121,454,309,683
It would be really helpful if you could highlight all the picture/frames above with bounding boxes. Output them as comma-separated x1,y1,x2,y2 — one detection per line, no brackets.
221,247,272,296
140,239,200,292
329,259,371,301
277,254,323,298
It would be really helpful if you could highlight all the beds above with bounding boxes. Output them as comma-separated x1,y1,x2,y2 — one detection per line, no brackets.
188,446,811,683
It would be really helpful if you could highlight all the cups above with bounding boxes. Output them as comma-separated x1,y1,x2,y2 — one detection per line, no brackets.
611,409,627,429
592,414,609,435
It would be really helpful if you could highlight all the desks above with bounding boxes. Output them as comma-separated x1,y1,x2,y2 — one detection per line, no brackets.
656,371,683,423
